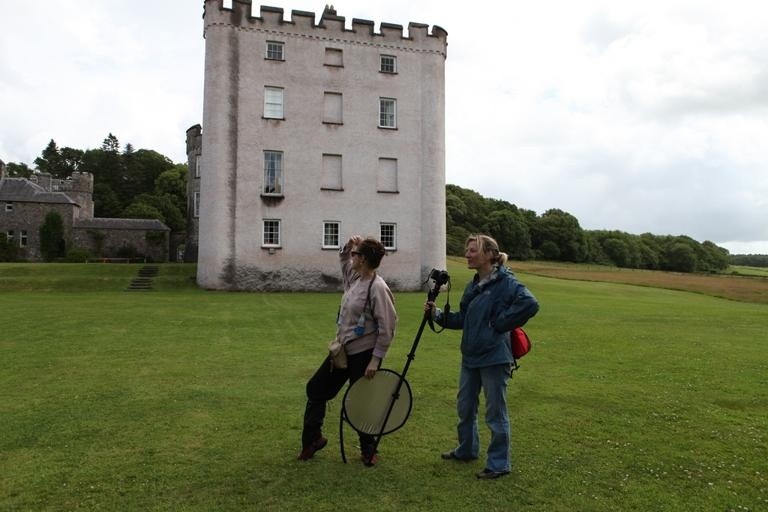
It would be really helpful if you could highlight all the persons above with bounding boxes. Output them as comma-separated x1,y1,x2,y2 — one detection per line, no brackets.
423,232,539,480
298,233,399,468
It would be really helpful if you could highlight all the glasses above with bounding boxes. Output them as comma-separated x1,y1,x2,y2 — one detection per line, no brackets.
351,251,360,257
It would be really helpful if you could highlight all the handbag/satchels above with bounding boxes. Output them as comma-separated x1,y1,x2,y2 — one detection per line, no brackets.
511,327,531,359
328,341,348,369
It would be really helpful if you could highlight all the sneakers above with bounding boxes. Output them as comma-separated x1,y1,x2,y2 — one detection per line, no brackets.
476,469,510,480
297,437,327,460
364,454,376,466
442,452,456,460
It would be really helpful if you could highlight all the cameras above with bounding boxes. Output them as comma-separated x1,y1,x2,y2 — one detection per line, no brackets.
430,269,449,285
